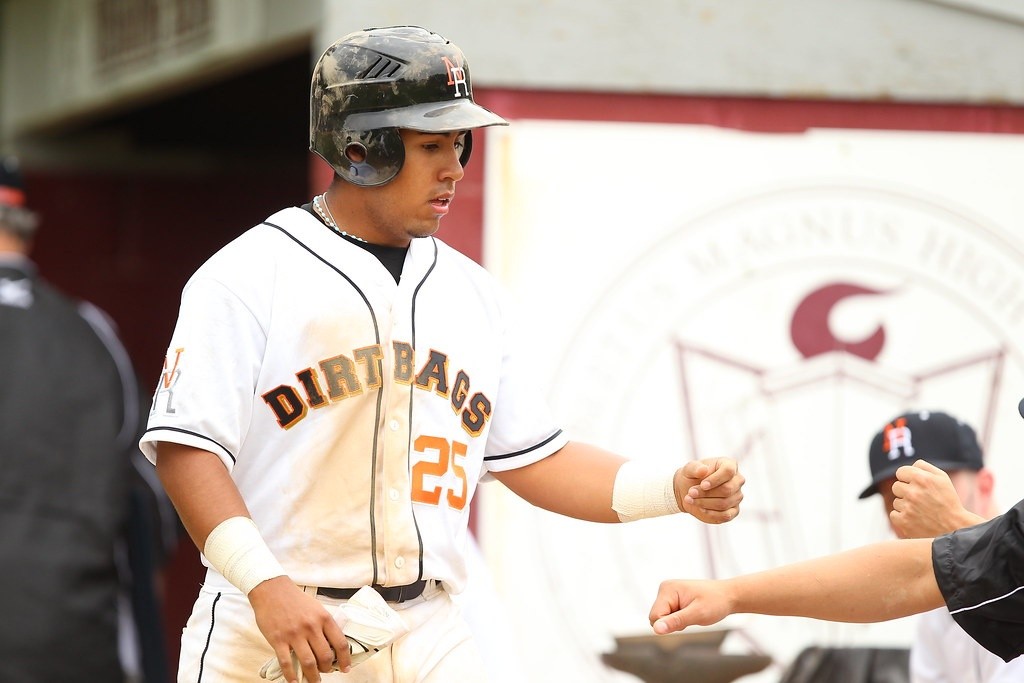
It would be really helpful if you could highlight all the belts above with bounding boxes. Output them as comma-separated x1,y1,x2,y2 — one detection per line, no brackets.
315,578,444,604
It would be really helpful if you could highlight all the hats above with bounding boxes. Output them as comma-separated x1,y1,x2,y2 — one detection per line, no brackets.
859,412,984,500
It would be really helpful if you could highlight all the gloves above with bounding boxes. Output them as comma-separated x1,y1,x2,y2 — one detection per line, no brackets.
259,585,410,683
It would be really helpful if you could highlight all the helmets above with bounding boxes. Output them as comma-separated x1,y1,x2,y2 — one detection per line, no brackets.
309,24,511,185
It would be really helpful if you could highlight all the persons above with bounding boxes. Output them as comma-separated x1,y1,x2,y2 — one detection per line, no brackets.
139,24,744,682
0,170,174,683
857,413,1024,682
649,397,1024,664
